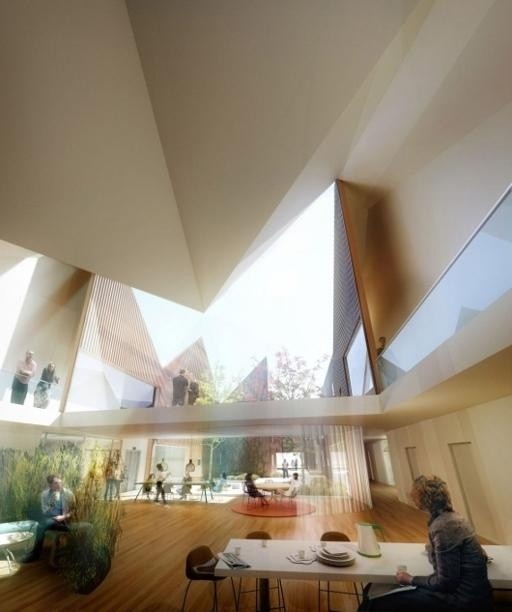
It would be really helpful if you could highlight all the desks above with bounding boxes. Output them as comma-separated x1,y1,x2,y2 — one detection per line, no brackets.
135,481,209,504
0,531,34,576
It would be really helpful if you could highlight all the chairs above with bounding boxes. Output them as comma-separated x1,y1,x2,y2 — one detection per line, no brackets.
318,532,364,612
180,545,238,612
278,485,300,507
235,531,287,612
241,481,259,510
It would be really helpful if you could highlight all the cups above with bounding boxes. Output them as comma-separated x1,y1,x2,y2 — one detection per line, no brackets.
398,565,407,586
234,547,241,556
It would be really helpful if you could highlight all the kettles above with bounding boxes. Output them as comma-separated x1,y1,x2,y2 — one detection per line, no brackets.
355,523,383,557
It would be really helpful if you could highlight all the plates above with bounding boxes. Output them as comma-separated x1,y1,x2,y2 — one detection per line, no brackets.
315,545,355,567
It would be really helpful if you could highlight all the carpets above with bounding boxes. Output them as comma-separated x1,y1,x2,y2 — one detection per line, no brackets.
231,499,317,517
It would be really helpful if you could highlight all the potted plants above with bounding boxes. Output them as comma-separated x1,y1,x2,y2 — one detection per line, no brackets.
62,440,124,595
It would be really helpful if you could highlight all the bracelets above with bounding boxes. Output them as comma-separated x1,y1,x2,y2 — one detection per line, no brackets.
64,514,67,520
409,576,414,585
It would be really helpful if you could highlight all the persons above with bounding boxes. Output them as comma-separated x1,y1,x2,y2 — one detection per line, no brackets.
245,473,269,505
11,351,37,403
189,379,199,406
21,475,75,562
277,453,299,496
143,458,227,505
376,336,397,387
34,362,59,407
104,461,122,502
362,475,492,610
172,369,187,404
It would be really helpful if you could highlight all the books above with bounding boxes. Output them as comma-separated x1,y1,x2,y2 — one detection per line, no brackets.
217,552,250,570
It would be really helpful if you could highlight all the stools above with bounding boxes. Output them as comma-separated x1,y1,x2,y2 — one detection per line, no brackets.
44,521,93,569
0,521,38,567
